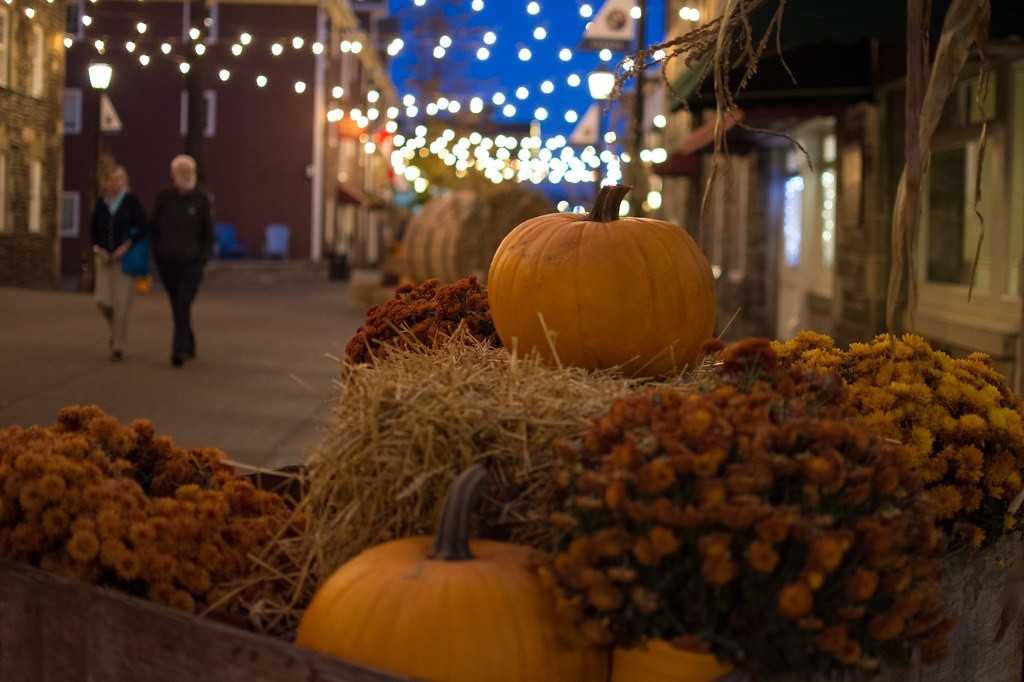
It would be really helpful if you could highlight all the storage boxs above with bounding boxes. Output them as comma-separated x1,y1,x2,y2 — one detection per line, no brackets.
0,457,1023,682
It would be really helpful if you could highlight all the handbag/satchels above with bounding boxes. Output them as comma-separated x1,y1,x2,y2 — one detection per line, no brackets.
119,229,151,277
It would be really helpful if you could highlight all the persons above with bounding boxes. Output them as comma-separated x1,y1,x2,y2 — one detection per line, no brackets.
147,154,214,369
90,161,146,363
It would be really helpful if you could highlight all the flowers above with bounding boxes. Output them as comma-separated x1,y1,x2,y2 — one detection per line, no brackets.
0,254,1023,682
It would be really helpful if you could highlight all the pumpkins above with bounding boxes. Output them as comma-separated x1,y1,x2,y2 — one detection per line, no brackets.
294,465,614,682
487,184,719,381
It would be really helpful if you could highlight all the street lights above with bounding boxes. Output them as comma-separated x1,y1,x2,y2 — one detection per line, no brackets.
84,53,115,291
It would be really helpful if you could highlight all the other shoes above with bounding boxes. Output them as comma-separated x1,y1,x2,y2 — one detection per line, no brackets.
109,352,121,362
170,354,184,368
